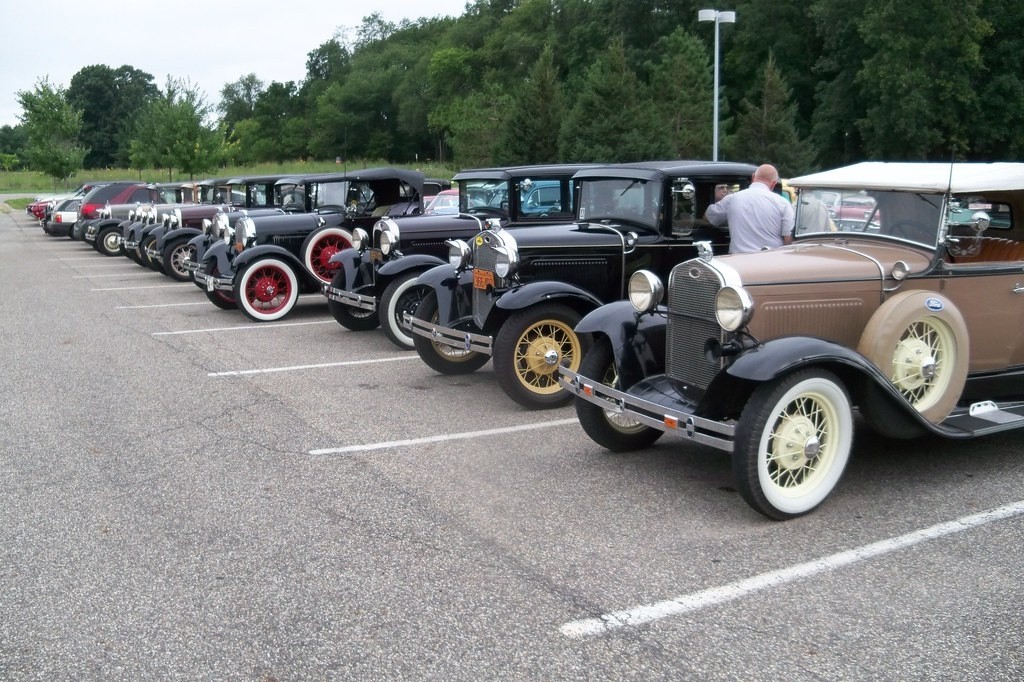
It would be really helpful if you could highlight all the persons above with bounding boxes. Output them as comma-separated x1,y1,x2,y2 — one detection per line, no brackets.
703,164,794,252
791,186,837,236
715,184,727,201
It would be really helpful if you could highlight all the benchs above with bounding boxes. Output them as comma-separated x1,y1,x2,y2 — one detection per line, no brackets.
945,235,1024,264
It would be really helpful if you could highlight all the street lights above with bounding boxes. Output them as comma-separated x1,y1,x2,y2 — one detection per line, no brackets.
698,9,736,161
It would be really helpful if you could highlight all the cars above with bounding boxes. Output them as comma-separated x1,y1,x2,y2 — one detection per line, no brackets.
399,157,783,411
25,164,1011,352
559,159,1024,522
